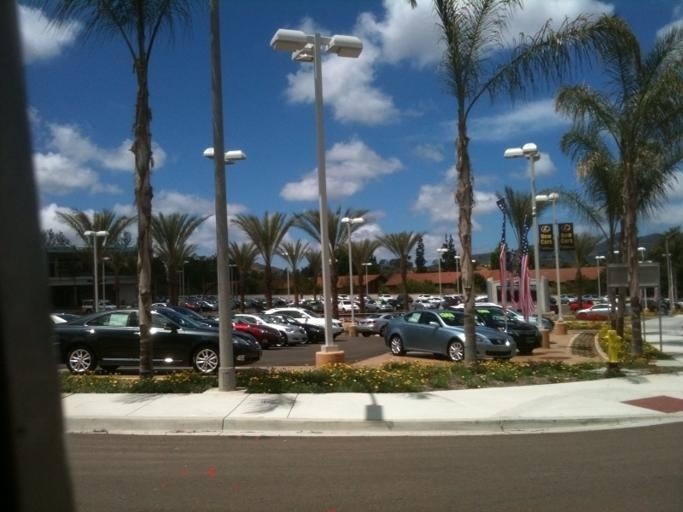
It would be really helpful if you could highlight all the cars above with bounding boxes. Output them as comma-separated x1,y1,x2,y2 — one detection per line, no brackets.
560,294,683,320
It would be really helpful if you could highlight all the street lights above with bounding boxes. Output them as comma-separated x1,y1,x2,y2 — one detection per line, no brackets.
502,142,543,325
229,263,237,295
202,147,247,164
82,230,110,312
594,245,647,309
182,260,189,296
267,29,372,347
436,246,461,300
534,193,563,321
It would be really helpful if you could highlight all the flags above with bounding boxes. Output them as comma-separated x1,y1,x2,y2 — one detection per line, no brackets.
519,219,535,316
498,212,508,309
